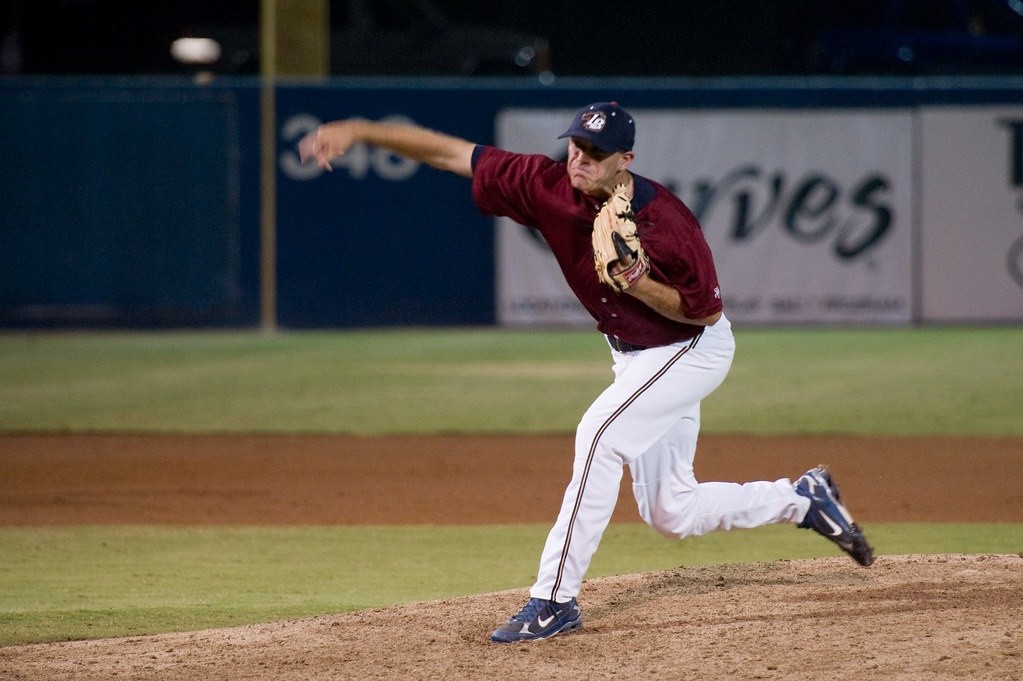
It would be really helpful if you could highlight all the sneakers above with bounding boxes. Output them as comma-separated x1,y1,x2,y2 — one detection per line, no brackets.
491,597,582,645
791,465,874,566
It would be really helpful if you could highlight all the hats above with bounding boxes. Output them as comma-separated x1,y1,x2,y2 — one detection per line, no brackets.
557,102,635,154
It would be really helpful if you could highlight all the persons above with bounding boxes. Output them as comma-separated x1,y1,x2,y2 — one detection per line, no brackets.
299,101,877,643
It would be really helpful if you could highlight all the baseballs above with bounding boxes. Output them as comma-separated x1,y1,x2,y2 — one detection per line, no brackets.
171,36,222,63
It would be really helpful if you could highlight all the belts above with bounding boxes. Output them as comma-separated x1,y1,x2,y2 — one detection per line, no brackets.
607,334,647,354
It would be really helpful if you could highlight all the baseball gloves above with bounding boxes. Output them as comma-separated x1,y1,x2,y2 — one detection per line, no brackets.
592,184,650,291
